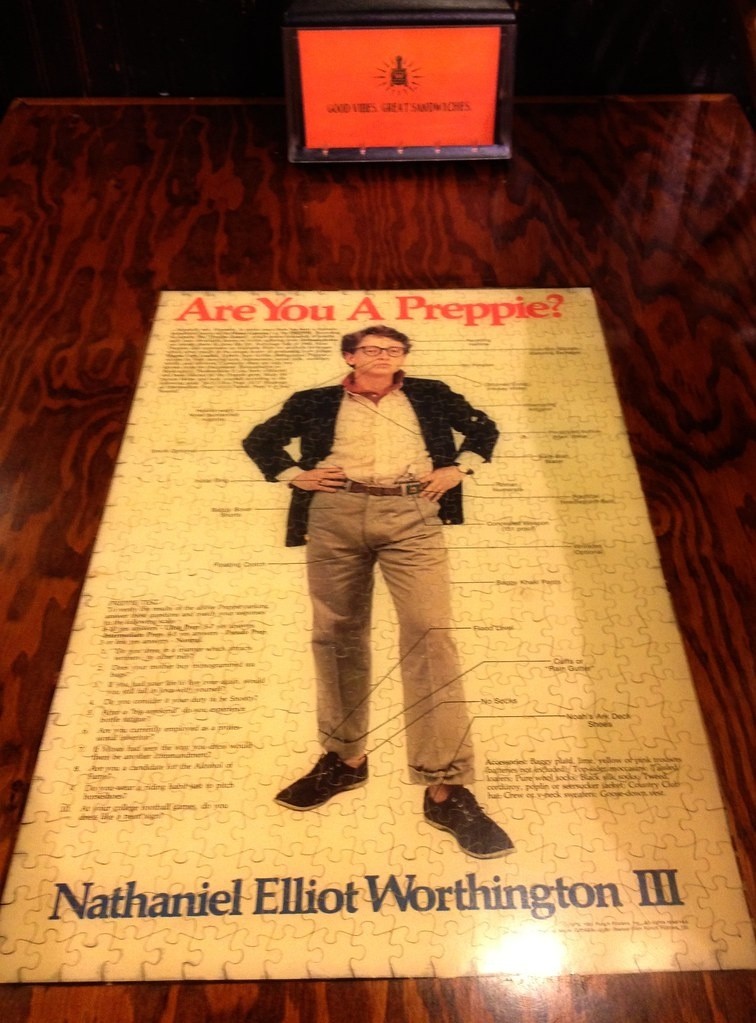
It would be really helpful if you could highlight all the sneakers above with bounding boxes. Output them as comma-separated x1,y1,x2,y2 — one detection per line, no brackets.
423,784,517,859
273,751,369,810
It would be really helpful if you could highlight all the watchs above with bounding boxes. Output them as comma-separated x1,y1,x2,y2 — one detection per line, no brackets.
456,463,474,475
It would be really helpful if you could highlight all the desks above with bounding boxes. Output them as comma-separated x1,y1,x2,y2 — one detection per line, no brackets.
0,86,756,1023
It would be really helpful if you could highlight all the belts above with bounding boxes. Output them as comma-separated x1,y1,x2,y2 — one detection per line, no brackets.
343,478,431,496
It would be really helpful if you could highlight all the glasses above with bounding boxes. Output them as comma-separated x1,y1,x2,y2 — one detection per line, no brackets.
355,346,406,357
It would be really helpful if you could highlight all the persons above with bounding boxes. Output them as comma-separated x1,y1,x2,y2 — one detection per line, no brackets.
242,326,515,859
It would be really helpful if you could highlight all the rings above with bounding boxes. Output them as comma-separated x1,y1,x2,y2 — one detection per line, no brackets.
318,481,323,485
